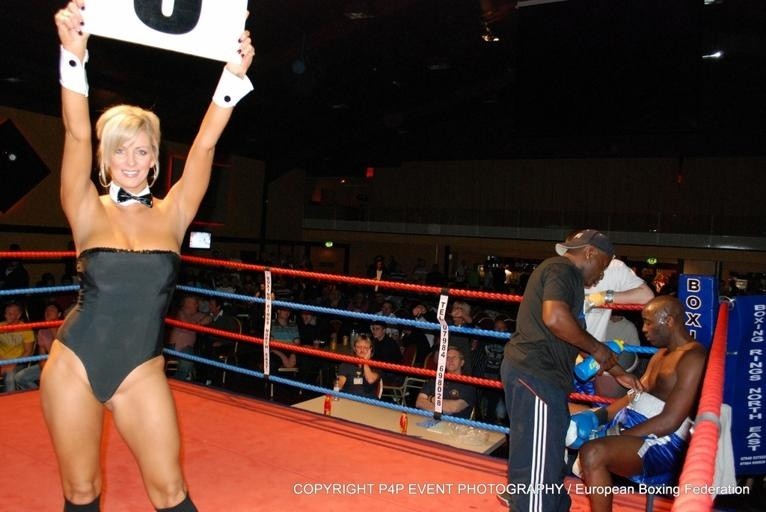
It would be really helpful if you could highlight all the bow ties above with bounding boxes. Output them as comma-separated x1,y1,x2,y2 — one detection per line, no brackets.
117,187,153,208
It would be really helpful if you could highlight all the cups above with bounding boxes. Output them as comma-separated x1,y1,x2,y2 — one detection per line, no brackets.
343,335,350,346
313,340,325,350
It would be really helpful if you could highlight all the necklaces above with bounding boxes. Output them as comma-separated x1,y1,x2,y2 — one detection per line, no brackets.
108,182,153,209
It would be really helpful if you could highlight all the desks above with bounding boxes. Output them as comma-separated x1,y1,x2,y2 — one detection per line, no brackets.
292,395,507,456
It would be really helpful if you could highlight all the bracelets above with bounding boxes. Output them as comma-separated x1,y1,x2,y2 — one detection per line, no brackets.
210,66,258,111
57,45,91,97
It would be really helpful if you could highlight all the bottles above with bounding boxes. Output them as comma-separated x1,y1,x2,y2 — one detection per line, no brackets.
324,394,331,418
334,375,340,401
350,330,359,355
330,333,337,352
573,339,624,381
400,409,408,437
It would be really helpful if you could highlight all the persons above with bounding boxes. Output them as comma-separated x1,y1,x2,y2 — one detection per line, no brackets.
38,1,258,511
0,257,765,433
496,296,712,512
567,233,655,381
497,227,614,512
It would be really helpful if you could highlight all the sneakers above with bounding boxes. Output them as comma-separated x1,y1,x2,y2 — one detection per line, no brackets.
496,491,512,507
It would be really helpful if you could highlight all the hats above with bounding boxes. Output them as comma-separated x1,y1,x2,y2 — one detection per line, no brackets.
555,229,616,260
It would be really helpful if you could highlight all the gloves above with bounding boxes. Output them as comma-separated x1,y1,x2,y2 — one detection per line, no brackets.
565,406,624,449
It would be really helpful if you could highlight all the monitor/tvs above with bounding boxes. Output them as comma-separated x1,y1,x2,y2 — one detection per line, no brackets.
188,229,214,251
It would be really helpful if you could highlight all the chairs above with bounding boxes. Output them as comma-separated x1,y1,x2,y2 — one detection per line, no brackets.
204,315,476,421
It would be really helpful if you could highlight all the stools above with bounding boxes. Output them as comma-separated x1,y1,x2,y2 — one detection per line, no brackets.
628,470,678,512
